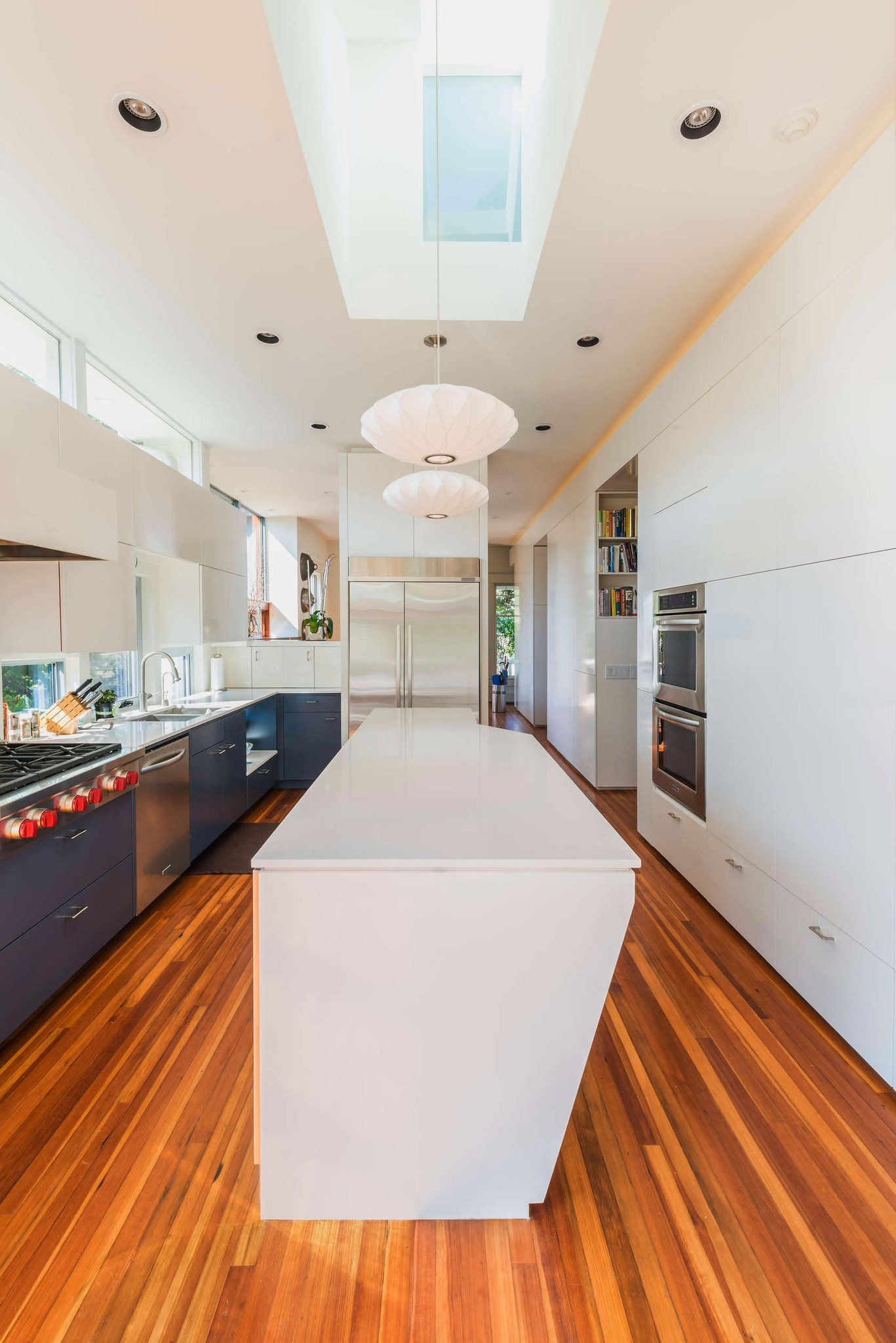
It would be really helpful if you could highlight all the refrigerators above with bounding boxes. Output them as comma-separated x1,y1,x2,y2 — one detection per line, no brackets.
349,581,480,738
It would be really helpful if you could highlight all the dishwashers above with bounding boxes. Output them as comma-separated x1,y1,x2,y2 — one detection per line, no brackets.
134,734,191,918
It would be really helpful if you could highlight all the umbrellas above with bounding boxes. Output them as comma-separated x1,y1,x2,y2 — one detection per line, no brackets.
491,656,508,685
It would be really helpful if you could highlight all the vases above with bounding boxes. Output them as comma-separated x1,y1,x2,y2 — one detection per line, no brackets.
95,703,114,721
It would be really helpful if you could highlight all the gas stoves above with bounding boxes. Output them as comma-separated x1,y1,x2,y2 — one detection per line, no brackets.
0,741,122,796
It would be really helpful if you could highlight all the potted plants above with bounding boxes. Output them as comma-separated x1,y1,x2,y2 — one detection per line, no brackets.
303,610,333,641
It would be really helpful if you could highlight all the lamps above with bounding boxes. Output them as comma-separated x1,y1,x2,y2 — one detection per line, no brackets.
382,334,489,519
360,1,521,465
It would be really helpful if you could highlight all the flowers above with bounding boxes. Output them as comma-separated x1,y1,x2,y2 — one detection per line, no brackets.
94,689,118,707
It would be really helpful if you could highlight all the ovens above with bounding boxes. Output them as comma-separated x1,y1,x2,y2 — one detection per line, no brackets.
651,583,706,823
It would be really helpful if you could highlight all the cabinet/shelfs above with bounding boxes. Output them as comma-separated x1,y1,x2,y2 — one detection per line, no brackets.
0,364,247,655
212,640,341,688
651,787,895,1089
594,490,638,619
187,710,247,863
0,790,136,1051
276,694,341,789
247,750,278,810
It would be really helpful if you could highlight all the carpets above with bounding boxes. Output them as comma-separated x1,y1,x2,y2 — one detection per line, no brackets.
186,822,279,874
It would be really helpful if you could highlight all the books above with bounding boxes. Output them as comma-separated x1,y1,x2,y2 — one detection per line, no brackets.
599,542,637,573
598,505,637,537
599,585,637,616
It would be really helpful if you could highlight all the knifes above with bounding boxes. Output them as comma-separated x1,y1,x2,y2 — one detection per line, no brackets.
71,678,104,709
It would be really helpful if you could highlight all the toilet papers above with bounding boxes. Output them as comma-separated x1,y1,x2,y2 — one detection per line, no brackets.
210,658,225,690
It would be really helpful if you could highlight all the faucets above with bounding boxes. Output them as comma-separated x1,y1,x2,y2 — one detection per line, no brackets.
139,651,181,712
160,671,175,706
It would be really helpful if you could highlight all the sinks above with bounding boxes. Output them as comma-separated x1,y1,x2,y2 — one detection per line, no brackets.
127,715,202,721
152,706,222,715
